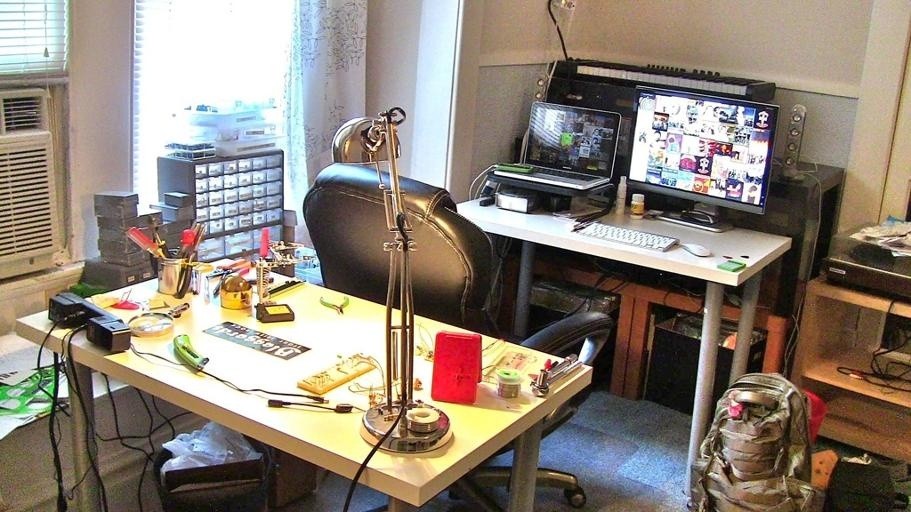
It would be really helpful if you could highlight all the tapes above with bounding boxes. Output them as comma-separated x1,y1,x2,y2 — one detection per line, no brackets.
495,366,523,384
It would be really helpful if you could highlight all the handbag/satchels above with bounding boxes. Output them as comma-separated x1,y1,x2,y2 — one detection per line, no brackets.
821,461,895,512
690,371,824,512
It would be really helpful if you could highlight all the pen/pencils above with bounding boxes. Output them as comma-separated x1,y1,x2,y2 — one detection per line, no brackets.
198,272,201,294
571,218,600,232
213,280,223,295
205,276,209,301
536,360,559,376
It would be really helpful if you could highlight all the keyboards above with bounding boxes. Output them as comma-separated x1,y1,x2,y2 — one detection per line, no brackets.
579,221,680,252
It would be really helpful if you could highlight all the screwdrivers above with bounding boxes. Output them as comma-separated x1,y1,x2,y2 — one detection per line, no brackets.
149,216,171,259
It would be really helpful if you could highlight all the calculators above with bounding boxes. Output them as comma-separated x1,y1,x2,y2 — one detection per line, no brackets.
718,259,746,272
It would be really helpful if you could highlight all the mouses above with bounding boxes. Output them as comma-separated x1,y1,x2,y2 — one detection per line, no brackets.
678,242,709,257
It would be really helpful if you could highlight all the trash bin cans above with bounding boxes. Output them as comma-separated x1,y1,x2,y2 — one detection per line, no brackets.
152,434,273,512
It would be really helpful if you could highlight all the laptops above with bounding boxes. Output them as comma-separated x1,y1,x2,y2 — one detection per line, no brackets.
494,102,621,189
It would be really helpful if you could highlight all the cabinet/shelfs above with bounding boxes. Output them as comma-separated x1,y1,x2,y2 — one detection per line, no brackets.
793,277,910,464
157,149,286,265
501,252,790,411
760,154,845,320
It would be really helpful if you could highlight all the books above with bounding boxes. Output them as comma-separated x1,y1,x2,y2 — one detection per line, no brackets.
0,331,62,442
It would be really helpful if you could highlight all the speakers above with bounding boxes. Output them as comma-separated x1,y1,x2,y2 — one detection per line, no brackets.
782,104,807,177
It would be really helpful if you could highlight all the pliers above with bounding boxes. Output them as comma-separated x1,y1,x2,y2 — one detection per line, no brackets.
320,296,350,315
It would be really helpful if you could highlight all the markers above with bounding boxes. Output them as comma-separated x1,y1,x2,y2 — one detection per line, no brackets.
192,268,197,294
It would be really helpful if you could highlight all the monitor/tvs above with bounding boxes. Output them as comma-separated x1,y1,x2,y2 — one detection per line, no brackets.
871,178,911,363
624,84,780,235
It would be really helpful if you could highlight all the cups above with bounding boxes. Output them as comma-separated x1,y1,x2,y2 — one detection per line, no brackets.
157,248,197,296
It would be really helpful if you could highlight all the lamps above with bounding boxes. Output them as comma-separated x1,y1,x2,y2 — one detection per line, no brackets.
545,0,584,105
333,105,452,453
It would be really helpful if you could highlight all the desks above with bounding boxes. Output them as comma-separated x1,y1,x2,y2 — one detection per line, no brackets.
17,255,595,512
458,197,793,496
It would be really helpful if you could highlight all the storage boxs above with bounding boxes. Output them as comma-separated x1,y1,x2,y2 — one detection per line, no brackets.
79,192,194,291
646,316,768,426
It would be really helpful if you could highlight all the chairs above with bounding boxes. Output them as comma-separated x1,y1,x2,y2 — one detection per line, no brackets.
303,165,615,512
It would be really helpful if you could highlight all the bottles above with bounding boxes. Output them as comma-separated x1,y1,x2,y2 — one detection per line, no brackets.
630,193,645,218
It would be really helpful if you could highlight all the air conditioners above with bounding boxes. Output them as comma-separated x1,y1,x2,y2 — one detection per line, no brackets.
0,88,60,282
